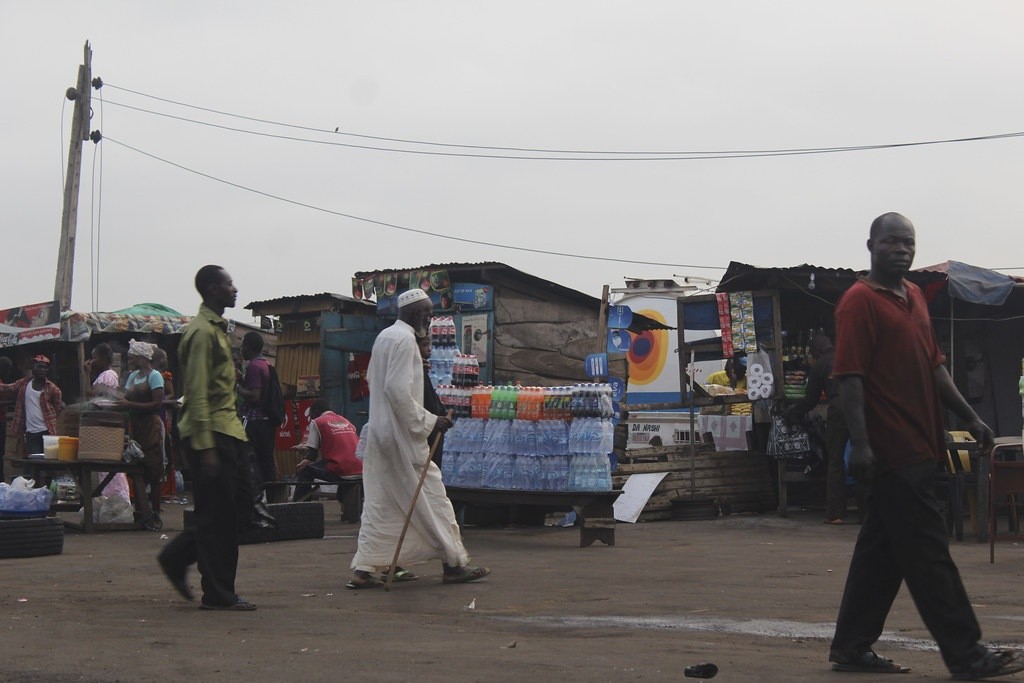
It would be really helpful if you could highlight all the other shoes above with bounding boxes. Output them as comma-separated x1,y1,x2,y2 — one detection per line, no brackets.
155,554,195,601
822,516,843,525
199,598,257,610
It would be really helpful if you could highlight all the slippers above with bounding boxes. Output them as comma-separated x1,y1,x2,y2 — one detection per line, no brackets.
345,576,385,588
381,571,418,581
950,649,1024,680
444,566,491,582
832,651,911,673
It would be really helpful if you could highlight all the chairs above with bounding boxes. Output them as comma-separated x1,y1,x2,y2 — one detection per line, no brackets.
935,431,1019,543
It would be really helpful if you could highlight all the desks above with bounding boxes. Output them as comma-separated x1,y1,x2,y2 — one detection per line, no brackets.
987,436,1024,563
11,457,156,533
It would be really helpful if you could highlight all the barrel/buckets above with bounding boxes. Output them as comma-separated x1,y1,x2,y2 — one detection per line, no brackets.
43,435,69,460
58,436,79,461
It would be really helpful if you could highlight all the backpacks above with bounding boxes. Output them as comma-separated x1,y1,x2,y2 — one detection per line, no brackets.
250,360,285,423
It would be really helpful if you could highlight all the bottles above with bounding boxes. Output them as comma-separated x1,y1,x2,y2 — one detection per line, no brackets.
571,383,613,418
517,387,545,421
488,380,522,419
471,380,492,420
451,353,479,386
50,478,81,505
429,316,456,346
514,453,569,491
510,419,537,456
435,384,472,418
485,419,512,455
569,416,614,454
537,418,569,455
347,352,362,402
543,387,573,421
483,452,515,490
427,345,461,389
441,452,485,488
442,417,487,453
569,453,612,492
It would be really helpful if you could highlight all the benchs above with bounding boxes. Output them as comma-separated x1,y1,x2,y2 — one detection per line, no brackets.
443,483,625,546
258,479,364,526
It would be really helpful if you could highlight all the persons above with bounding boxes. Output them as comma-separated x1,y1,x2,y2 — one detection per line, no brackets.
156,265,256,611
698,356,752,418
828,213,1024,680
0,339,178,514
234,288,491,588
782,334,865,524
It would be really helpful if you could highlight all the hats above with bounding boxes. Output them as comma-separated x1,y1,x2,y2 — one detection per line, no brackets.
31,355,50,367
397,289,429,308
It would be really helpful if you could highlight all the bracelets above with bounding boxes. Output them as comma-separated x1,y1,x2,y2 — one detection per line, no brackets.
235,384,240,391
